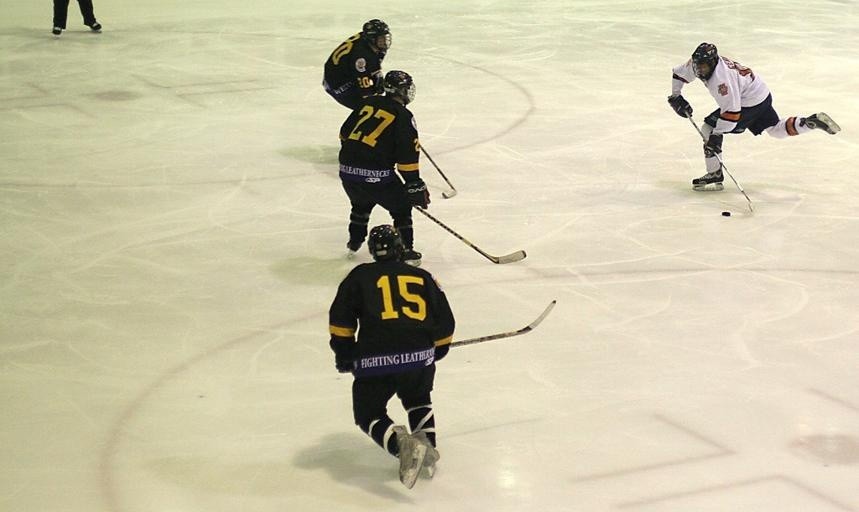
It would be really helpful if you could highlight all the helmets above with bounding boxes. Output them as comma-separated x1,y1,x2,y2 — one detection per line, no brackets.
384,71,415,104
363,20,391,50
691,43,717,80
369,225,402,258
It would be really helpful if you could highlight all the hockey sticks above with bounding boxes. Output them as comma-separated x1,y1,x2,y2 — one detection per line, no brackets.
419,145,457,199
683,111,755,217
412,204,526,264
448,300,556,347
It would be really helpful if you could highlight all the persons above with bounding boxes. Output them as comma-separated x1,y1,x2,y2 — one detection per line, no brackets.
52,0,102,36
338,70,430,262
668,42,841,185
322,18,393,110
328,225,456,486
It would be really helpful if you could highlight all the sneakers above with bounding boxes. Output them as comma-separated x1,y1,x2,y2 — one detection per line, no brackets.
403,250,421,260
804,114,837,134
393,426,439,488
692,170,724,186
347,239,361,250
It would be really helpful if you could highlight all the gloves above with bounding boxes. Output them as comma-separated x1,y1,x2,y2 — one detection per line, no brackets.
669,95,693,118
407,180,430,208
703,133,723,158
